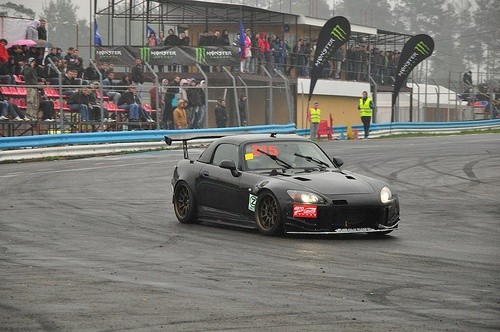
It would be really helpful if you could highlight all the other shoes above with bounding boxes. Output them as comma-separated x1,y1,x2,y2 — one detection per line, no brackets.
364,135,368,139
0,116,9,120
44,119,55,122
104,117,116,122
147,117,155,123
24,115,30,121
14,115,22,121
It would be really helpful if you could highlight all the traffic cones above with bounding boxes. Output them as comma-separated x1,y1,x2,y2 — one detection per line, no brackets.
353,128,359,139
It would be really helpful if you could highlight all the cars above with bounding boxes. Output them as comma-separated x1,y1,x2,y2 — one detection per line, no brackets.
163,131,400,236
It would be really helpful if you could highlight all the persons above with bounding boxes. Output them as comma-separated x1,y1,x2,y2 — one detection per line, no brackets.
458,71,499,114
0,0,402,131
308,101,321,142
268,144,296,168
358,91,375,139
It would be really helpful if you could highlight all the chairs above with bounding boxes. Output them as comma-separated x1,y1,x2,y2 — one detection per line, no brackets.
317,120,332,141
0,75,157,137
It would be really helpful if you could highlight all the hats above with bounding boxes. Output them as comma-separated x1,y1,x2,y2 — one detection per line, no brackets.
314,101,319,104
